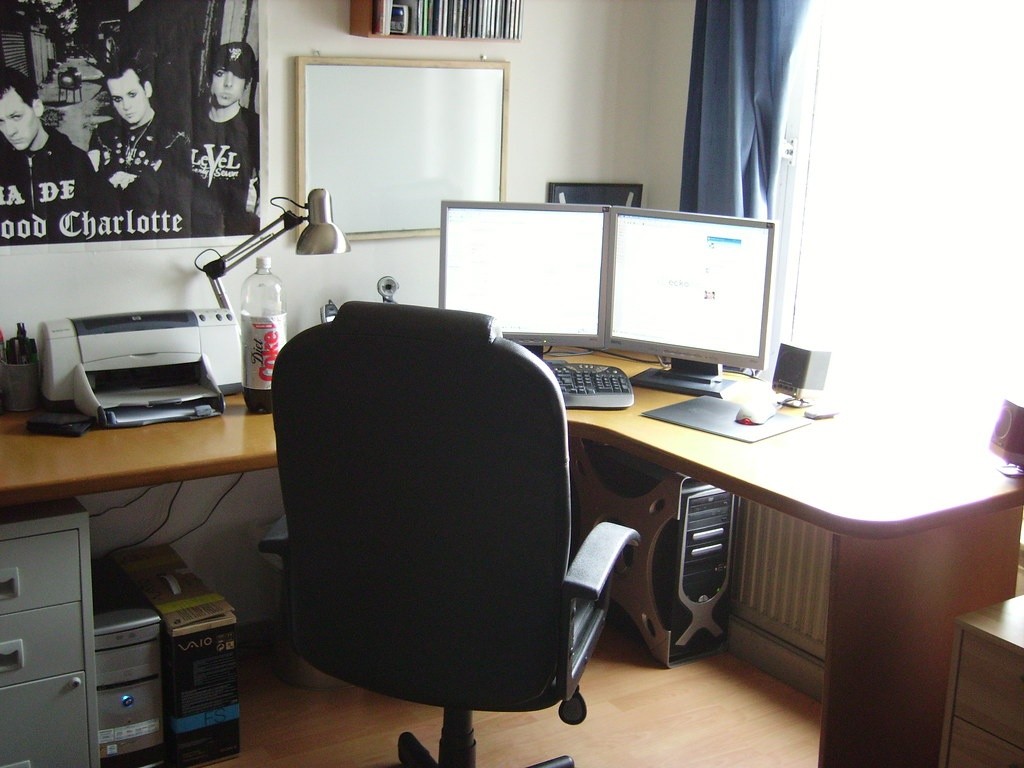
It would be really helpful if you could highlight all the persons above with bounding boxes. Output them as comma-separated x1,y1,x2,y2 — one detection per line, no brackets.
0,42,260,244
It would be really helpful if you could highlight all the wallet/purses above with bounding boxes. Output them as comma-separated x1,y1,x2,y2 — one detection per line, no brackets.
26,412,97,436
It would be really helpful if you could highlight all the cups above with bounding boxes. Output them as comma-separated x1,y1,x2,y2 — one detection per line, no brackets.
0,358,42,412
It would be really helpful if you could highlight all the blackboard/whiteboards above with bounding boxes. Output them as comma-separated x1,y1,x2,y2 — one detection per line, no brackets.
295,54,511,241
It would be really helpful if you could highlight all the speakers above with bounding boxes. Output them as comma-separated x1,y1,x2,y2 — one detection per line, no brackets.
771,343,831,409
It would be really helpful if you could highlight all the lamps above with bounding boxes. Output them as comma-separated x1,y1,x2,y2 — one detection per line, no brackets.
193,188,352,309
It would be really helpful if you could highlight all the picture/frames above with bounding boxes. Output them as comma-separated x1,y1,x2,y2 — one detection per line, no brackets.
291,50,511,241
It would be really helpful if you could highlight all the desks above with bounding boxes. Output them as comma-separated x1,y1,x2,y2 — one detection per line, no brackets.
0,340,1024,768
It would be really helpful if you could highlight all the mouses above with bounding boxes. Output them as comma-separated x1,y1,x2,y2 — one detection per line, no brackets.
734,398,776,425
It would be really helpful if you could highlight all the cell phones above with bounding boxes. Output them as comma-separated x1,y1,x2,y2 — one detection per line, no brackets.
805,409,839,420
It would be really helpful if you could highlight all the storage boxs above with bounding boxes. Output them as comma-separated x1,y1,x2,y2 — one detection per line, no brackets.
120,543,241,768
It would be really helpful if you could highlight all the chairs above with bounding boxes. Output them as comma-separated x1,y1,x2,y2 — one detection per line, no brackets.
255,300,644,768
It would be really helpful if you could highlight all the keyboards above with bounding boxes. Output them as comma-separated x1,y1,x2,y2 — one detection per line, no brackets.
544,362,635,408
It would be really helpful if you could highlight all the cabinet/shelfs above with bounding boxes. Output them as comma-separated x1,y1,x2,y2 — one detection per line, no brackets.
0,498,101,768
937,594,1024,768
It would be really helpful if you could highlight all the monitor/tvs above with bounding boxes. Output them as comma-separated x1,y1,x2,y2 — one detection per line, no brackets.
440,200,610,363
605,206,776,399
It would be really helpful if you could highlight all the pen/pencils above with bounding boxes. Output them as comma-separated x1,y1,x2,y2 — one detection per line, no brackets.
0,322,38,365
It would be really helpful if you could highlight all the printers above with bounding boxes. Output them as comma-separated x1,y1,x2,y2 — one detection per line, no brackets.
35,306,246,430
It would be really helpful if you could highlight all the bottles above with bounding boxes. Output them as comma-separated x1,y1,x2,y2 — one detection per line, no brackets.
238,256,287,415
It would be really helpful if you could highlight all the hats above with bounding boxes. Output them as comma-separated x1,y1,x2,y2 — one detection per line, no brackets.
214,42,256,79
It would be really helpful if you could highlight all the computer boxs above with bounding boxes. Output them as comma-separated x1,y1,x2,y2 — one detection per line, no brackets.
571,434,742,669
89,553,168,768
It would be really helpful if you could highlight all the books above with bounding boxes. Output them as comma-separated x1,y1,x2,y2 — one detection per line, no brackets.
364,0,523,41
